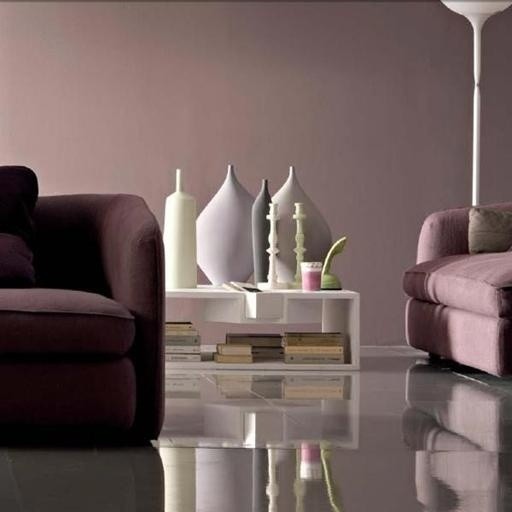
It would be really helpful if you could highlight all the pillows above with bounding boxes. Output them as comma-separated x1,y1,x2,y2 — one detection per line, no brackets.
1,166,39,288
468,208,510,255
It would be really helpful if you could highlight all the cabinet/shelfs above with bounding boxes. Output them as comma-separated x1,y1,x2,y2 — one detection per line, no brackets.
155,370,360,452
165,283,363,371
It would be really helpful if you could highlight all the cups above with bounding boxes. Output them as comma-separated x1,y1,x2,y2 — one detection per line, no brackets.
299,442,322,480
300,262,323,292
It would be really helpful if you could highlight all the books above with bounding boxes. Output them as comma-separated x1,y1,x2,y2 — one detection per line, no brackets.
214,372,342,399
165,322,201,362
166,370,202,398
211,327,346,364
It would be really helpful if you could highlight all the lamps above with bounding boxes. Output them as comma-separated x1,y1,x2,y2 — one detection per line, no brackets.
440,0,512,209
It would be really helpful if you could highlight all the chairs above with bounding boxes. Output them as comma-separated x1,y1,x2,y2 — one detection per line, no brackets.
403,201,510,380
404,361,512,512
0,166,167,453
